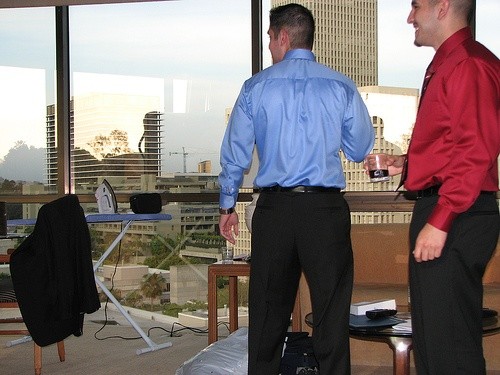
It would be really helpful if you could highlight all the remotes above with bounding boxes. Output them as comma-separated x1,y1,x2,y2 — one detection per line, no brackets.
366,309,398,320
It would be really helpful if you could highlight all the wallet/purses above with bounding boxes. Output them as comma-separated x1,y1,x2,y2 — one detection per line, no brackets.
129,192,162,213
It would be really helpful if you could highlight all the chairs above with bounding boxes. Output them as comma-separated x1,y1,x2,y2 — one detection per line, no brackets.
0,249,66,375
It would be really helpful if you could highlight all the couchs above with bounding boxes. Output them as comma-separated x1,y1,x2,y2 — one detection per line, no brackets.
301,222,500,375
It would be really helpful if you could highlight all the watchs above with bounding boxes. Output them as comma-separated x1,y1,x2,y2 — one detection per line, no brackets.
218,207,235,215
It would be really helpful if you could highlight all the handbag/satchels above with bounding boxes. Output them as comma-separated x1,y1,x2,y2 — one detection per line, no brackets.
279,329,319,374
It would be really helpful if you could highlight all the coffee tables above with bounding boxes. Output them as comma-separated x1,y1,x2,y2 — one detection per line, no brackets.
305,305,500,375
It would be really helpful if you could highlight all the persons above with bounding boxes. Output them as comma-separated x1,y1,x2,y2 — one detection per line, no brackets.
219,3,375,375
363,0,500,375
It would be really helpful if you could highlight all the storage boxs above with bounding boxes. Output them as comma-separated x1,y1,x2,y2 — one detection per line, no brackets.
350,298,397,316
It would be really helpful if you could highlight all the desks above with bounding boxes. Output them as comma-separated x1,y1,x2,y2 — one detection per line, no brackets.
6,214,174,355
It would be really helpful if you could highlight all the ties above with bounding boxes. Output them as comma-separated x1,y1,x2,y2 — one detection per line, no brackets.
394,61,433,191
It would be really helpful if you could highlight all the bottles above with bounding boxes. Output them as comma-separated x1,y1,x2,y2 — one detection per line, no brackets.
0,201,8,236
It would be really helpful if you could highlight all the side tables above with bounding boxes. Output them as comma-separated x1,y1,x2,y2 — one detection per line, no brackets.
207,261,301,346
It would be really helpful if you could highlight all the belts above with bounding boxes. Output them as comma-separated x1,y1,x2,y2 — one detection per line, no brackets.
260,184,341,193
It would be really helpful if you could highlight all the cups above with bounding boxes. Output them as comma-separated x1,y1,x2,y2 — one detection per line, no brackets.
221,247,234,264
363,153,389,183
